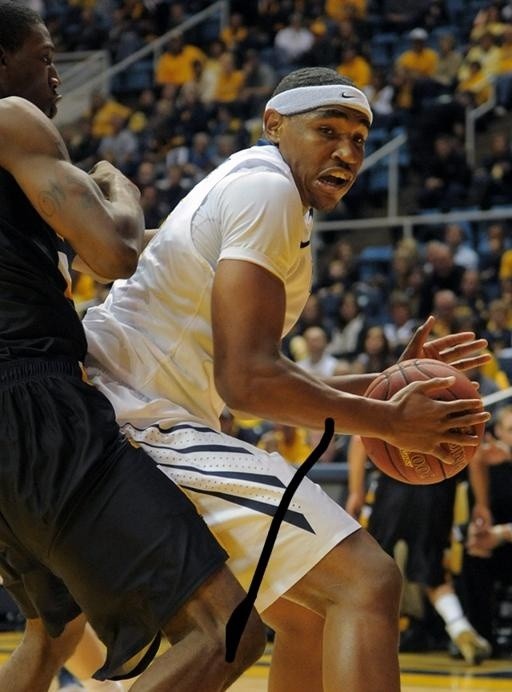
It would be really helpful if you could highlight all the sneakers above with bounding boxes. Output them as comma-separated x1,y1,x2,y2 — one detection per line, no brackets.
455,630,491,666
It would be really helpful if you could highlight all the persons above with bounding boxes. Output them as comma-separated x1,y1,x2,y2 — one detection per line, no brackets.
1,0,512,692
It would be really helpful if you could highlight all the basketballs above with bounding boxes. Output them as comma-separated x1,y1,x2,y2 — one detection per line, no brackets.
355,359,482,487
